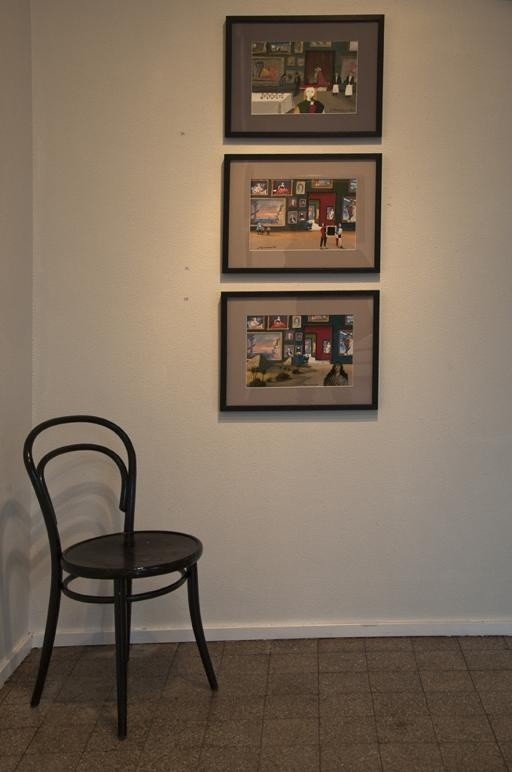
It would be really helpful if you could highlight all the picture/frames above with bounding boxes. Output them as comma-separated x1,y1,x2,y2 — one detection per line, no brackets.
225,14,385,139
220,290,379,412
221,153,381,274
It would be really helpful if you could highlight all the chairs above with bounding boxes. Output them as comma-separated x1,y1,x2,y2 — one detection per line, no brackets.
23,415,218,739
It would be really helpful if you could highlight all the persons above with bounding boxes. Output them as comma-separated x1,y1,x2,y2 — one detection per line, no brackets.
252,182,355,249
332,71,342,96
345,72,354,98
294,87,326,113
324,360,348,386
